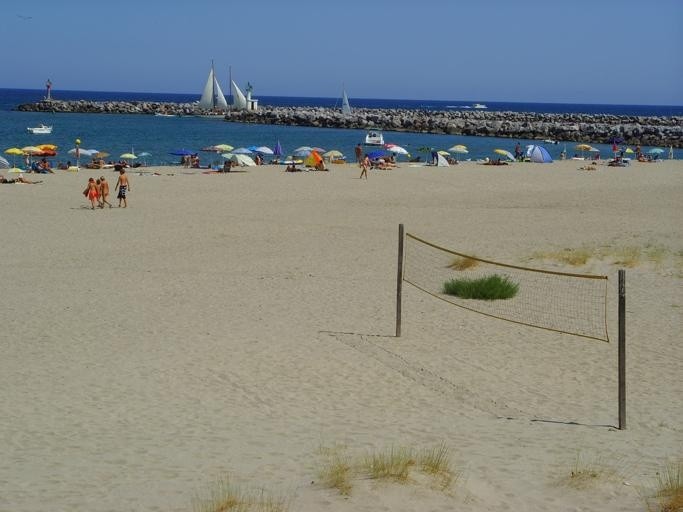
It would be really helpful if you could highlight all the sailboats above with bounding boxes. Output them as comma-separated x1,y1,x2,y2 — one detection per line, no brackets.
195,59,247,119
332,80,355,117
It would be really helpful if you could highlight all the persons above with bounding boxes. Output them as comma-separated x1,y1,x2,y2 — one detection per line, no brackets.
285,161,326,171
360,155,370,178
32,159,130,211
